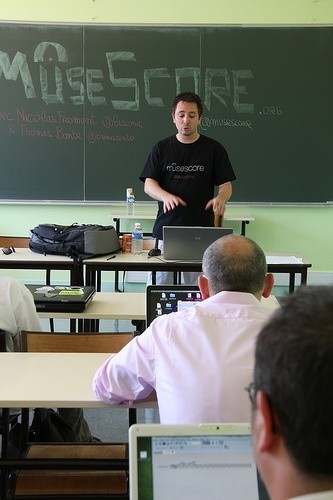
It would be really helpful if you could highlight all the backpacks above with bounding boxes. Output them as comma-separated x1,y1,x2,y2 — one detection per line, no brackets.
28,223,122,257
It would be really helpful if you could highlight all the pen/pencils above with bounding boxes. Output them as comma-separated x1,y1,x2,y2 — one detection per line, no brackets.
107,255,116,261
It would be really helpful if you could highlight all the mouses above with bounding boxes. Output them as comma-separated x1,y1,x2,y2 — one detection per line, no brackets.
148,249,161,257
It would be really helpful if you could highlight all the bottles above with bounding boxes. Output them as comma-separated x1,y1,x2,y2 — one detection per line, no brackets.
126,189,135,216
131,223,143,256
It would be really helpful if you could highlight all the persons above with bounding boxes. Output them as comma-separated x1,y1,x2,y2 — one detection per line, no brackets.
92,233,275,424
139,91,237,334
245,284,333,500
0,278,43,414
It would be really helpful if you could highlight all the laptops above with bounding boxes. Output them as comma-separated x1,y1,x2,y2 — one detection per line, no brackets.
145,285,203,330
163,226,233,261
128,423,271,500
24,284,96,311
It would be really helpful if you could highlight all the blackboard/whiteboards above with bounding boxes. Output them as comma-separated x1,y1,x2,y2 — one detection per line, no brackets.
0,19,333,209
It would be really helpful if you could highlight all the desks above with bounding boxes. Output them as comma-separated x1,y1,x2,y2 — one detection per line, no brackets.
84,252,310,332
0,247,85,333
0,352,158,500
36,293,282,332
111,212,256,293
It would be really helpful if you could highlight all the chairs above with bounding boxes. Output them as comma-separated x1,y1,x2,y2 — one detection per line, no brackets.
0,330,134,499
1,235,54,333
